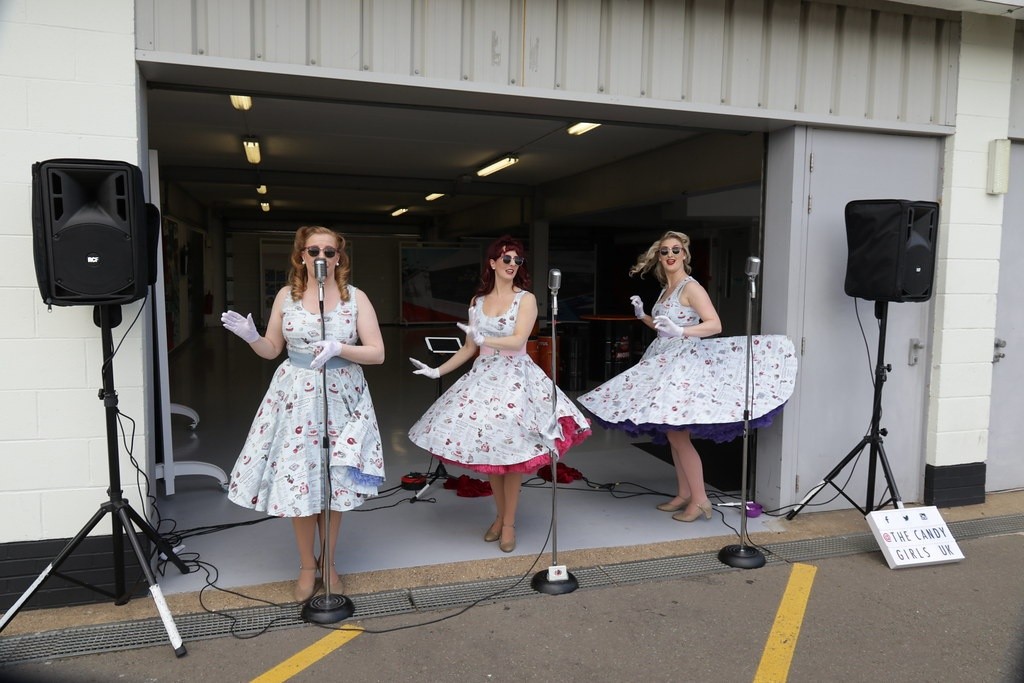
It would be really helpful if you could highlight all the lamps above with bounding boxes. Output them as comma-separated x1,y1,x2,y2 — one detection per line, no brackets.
566,121,602,137
230,94,254,111
243,140,261,167
424,191,445,200
392,207,408,217
257,183,267,196
259,199,271,213
477,154,519,176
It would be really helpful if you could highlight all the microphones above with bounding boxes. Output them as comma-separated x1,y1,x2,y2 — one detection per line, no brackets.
314,257,327,310
745,256,760,301
548,269,561,321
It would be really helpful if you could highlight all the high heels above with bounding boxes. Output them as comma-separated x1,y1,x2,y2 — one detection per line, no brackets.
317,554,343,594
672,499,712,521
500,522,515,552
294,553,318,602
657,494,693,512
485,514,502,542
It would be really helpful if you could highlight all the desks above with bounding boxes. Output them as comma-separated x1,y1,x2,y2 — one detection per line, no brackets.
546,319,586,377
580,315,638,363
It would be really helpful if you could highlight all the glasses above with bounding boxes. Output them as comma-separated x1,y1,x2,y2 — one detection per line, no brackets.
658,247,684,256
305,246,338,258
500,254,523,266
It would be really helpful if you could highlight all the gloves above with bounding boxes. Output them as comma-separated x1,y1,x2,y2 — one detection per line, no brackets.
630,295,644,319
653,315,683,337
310,340,342,369
410,358,439,379
457,321,484,346
221,310,259,343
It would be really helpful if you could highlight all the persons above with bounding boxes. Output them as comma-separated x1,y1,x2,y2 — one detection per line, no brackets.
217,225,385,605
576,231,798,522
405,238,594,554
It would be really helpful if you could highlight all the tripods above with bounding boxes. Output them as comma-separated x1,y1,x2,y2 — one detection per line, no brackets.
787,302,905,521
411,337,464,505
0,305,192,657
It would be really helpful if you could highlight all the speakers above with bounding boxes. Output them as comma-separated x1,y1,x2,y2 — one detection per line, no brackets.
845,200,940,305
30,157,149,307
